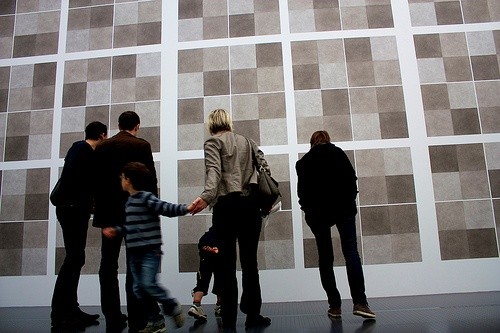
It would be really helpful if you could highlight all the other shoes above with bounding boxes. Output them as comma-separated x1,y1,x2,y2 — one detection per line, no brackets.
244,314,270,325
75,305,100,320
327,304,342,316
106,313,128,332
51,317,97,327
353,299,376,317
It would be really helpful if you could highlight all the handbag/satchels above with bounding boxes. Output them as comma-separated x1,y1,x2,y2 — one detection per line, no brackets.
50,178,73,207
243,136,282,216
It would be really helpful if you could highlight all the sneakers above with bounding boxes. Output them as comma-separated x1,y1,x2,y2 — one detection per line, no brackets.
138,314,166,333
169,310,186,331
214,303,222,314
187,302,207,319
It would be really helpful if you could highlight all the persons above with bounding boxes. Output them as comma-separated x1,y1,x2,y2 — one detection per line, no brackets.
49,120,108,328
92,111,158,333
190,109,271,333
187,214,222,320
103,162,201,333
295,130,376,319
